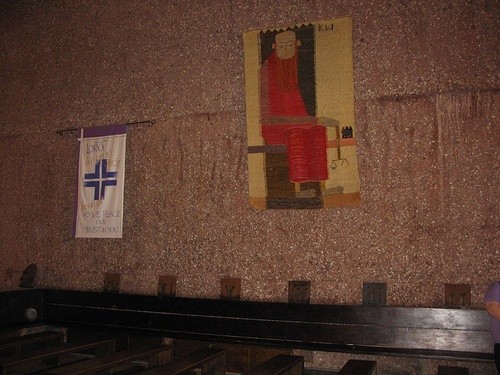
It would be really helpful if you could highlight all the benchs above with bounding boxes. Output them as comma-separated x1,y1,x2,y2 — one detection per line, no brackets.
134,346,225,375
236,354,304,375
0,330,62,363
0,335,117,375
337,359,378,375
37,342,177,375
438,366,469,375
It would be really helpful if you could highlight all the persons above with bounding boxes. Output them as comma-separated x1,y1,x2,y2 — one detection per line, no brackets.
484,279,500,375
